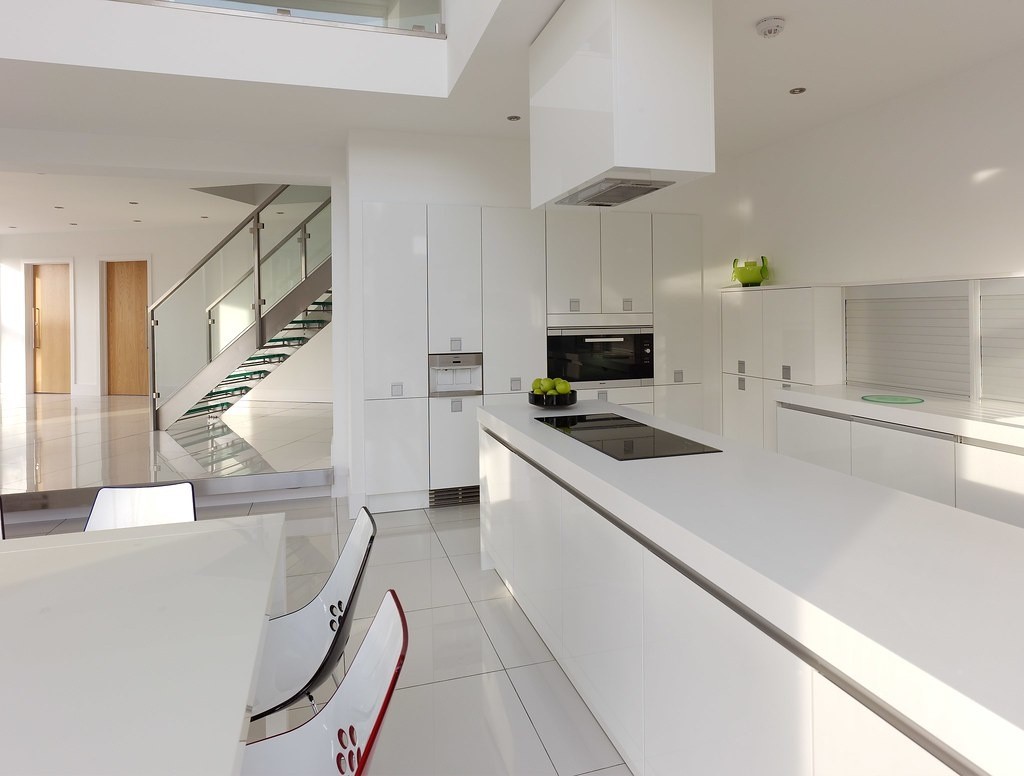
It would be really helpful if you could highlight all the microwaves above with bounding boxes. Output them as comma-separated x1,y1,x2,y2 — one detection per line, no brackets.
547,327,654,390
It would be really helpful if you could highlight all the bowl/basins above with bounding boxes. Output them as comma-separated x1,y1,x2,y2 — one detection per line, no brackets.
529,391,577,407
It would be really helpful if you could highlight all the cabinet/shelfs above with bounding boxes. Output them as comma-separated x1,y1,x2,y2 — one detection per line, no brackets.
652,210,706,386
653,383,703,429
850,415,956,507
482,205,547,395
426,206,484,356
603,436,655,460
428,396,484,490
777,406,851,475
364,203,430,496
762,282,843,386
717,286,762,377
956,442,1024,531
576,386,654,417
545,206,653,315
763,378,811,453
718,372,763,449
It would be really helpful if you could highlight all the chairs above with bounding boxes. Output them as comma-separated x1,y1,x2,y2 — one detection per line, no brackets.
240,588,408,776
248,504,379,725
82,481,197,535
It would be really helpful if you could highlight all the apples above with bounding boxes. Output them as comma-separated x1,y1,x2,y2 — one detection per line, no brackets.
532,378,570,395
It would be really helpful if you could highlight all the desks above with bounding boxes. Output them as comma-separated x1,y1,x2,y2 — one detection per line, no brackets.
1,510,289,776
